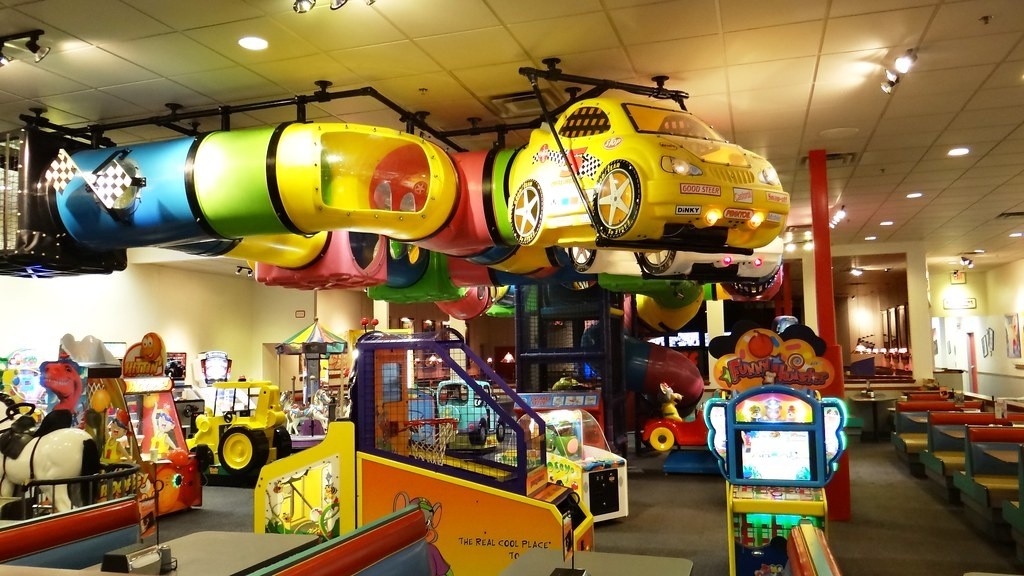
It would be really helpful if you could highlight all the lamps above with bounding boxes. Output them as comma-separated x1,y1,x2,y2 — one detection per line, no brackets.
247,269,252,277
0,42,14,67
880,77,899,95
25,36,51,64
952,270,958,277
895,47,919,74
960,257,973,269
234,267,241,274
330,0,348,10
293,0,317,13
885,69,897,82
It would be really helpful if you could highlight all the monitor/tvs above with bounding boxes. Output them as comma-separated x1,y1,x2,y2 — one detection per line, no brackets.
735,428,818,482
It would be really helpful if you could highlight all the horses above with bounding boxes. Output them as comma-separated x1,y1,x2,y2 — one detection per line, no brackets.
279,389,352,436
0,392,103,513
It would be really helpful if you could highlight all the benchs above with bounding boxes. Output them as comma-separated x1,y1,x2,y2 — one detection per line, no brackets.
844,390,1024,564
0,494,844,576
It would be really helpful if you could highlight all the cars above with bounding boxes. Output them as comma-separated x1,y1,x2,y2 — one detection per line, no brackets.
436,379,508,445
509,99,792,257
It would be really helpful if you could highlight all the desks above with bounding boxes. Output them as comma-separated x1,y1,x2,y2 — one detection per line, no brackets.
939,430,965,440
497,548,694,576
80,530,319,576
907,416,928,423
851,395,898,442
982,450,1018,465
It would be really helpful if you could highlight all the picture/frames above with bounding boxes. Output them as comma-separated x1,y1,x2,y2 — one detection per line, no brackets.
981,335,988,358
988,327,994,351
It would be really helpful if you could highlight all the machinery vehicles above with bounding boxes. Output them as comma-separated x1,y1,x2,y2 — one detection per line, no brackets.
179,380,296,483
